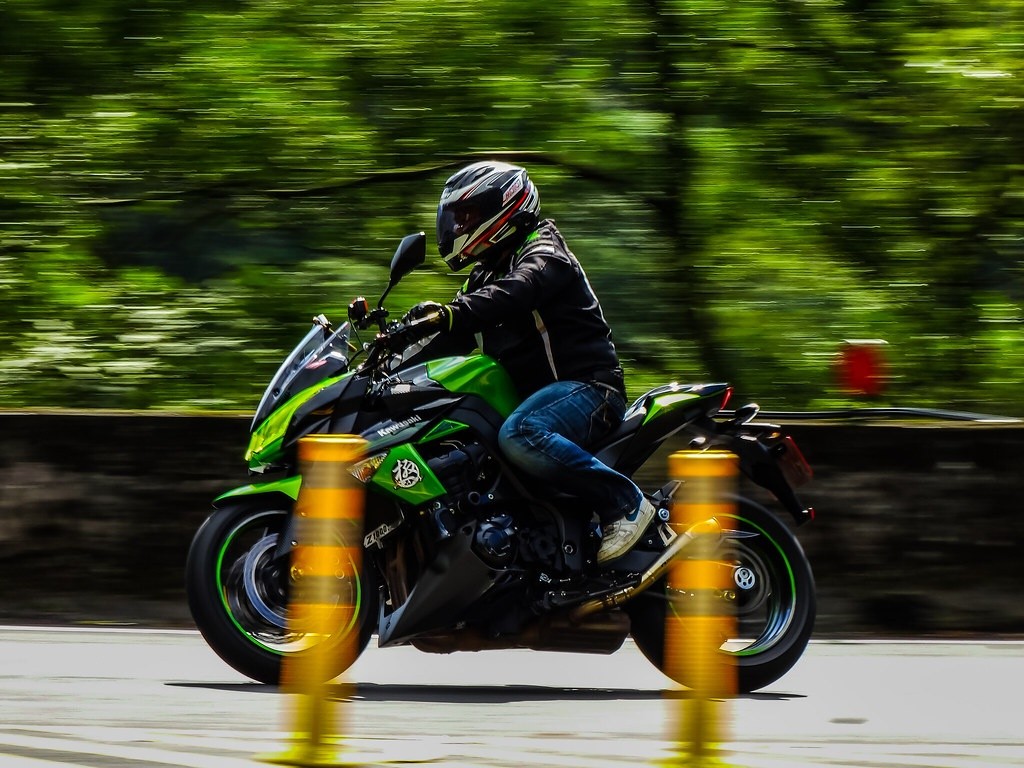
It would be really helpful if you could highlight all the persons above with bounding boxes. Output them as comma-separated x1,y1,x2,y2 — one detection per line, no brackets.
401,161,656,564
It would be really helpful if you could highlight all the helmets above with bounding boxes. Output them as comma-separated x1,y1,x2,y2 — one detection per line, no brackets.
437,161,540,271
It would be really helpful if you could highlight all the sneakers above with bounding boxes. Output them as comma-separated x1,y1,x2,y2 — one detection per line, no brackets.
596,494,656,562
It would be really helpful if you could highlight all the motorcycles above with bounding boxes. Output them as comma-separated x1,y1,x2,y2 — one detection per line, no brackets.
185,231,819,695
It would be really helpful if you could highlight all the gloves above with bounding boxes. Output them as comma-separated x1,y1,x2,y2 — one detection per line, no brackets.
402,301,452,332
